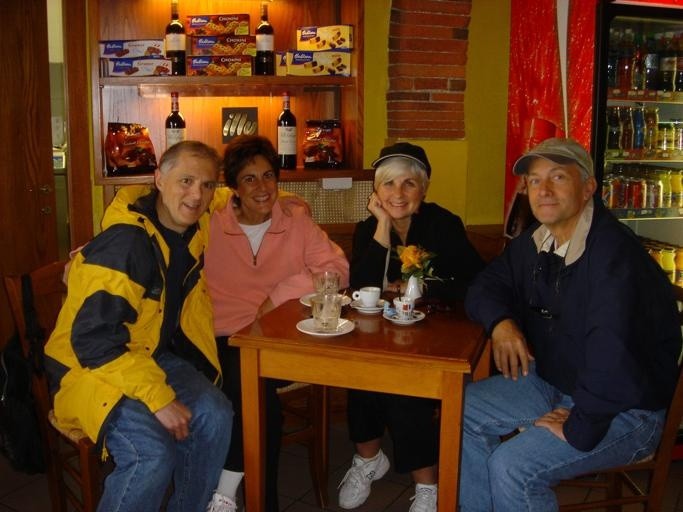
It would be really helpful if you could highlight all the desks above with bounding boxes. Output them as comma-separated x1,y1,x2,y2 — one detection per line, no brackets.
224,287,506,510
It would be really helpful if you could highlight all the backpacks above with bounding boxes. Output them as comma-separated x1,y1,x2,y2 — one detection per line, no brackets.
1,273,58,477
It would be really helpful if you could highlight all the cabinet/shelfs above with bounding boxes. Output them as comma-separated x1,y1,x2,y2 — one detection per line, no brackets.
81,2,367,193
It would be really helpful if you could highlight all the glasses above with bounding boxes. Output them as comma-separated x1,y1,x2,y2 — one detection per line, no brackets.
522,302,554,322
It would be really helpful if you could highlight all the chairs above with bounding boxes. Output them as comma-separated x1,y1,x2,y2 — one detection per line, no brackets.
544,275,683,512
4,252,120,512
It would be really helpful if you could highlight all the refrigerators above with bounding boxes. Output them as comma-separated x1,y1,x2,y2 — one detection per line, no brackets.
505,1,682,287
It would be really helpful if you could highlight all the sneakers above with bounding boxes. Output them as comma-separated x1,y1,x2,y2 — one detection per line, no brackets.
205,493,238,512
407,483,439,512
336,447,390,508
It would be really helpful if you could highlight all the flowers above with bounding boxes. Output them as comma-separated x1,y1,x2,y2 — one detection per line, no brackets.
392,239,435,283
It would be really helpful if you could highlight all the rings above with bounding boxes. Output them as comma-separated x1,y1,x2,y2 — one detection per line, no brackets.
368,195,372,200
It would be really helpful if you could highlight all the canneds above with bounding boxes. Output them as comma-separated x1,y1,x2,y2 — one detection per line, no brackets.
601,173,664,209
658,122,683,150
302,118,345,169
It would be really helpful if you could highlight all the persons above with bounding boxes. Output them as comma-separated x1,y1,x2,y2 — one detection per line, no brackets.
42,141,312,510
337,142,487,511
459,136,680,509
62,133,350,510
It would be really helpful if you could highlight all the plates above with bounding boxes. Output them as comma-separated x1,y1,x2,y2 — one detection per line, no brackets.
299,291,351,310
383,310,424,324
350,300,391,314
296,316,354,339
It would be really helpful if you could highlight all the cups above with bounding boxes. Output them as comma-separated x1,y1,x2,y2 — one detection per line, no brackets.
311,295,341,331
311,270,339,303
394,297,415,319
353,286,379,306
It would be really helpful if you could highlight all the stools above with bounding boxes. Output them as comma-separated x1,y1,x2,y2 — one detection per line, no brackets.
270,377,331,512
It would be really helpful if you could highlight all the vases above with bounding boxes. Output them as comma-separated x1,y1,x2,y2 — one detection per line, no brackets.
401,276,422,302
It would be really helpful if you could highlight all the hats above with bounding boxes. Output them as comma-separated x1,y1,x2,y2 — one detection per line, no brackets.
372,142,431,179
513,137,594,179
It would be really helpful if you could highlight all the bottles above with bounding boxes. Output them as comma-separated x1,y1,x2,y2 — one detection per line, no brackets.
255,4,275,77
275,90,300,171
604,22,683,284
164,0,186,75
163,92,185,149
